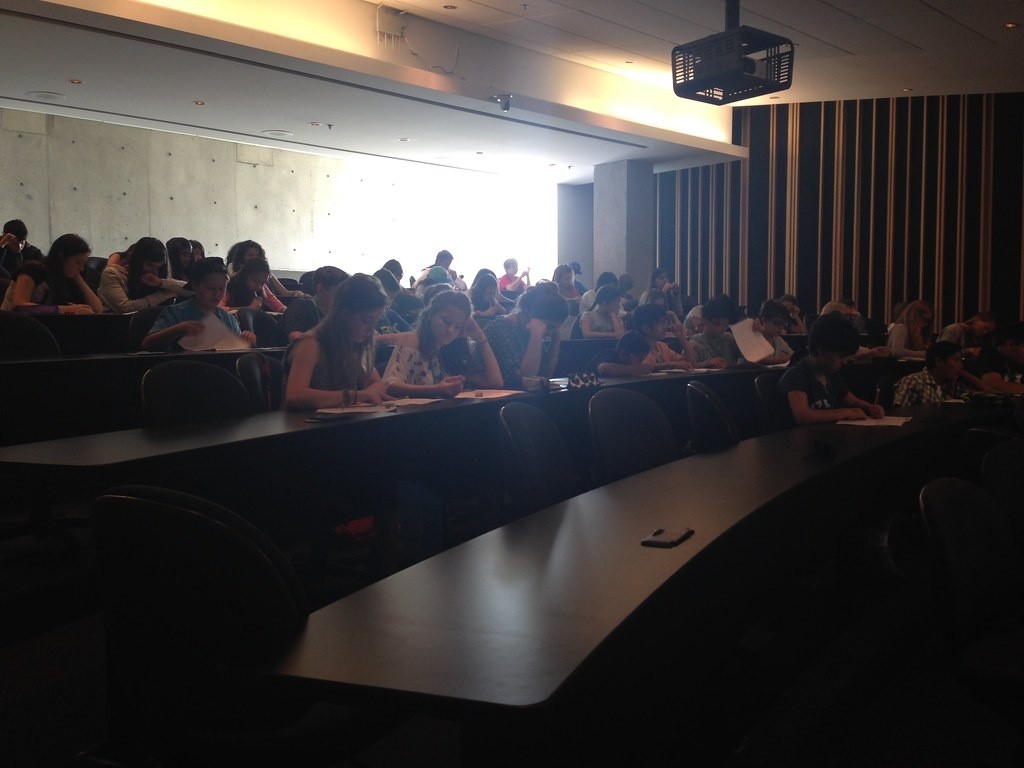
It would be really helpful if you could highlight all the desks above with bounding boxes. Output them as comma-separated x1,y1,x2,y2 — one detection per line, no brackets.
0,366,755,476
262,390,1015,720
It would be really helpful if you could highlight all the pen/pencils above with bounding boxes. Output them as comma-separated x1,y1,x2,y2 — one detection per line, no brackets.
781,349,791,355
874,387,880,405
65,301,96,314
254,289,258,298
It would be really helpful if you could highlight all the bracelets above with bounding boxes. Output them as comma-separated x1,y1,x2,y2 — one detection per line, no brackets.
342,388,357,404
478,336,489,344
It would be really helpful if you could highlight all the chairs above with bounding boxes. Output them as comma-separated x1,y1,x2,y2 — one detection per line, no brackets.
0,296,1021,767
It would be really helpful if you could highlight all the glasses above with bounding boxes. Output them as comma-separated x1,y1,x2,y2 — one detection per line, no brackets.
768,317,789,328
712,317,731,329
950,354,965,363
654,318,667,327
920,315,930,324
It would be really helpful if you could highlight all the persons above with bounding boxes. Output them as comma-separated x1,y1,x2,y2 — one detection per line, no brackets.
0,220,1024,439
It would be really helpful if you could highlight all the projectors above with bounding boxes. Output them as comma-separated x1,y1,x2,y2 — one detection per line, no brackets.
692,54,776,91
